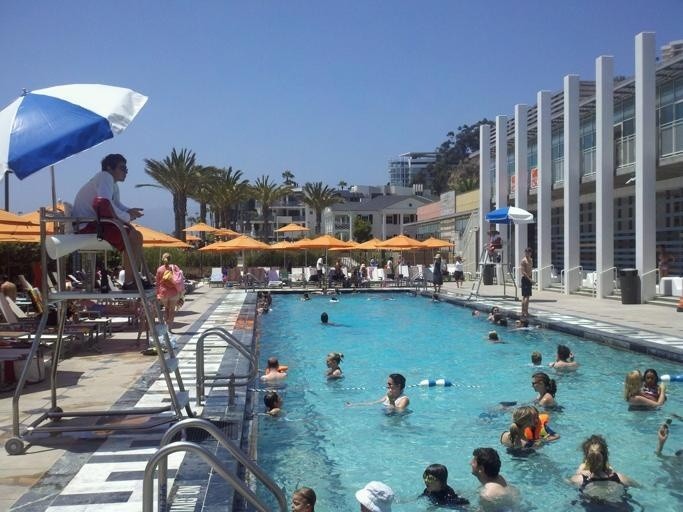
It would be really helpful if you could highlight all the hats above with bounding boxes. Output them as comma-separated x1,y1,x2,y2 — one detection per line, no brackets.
434,254,441,259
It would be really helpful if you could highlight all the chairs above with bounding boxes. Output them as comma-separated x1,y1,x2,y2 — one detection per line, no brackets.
490,239,505,262
60,201,113,291
0,269,199,390
210,263,475,289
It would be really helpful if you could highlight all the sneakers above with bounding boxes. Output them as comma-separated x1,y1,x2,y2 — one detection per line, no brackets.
123,280,154,293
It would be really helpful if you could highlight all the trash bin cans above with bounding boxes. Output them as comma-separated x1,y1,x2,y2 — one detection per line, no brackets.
482,264,494,285
619,268,638,304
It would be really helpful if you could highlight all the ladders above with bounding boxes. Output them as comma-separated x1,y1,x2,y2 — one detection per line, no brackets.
467,240,519,301
5,207,197,455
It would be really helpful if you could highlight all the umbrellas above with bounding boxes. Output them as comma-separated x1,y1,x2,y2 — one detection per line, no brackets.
0,84,148,325
485,205,534,272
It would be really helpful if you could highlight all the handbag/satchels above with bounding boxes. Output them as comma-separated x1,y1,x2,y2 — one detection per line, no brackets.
162,270,175,289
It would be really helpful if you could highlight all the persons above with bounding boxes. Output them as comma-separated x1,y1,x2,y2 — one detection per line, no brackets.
301,254,466,303
469,448,520,512
655,425,683,456
320,312,343,327
472,307,532,332
572,435,639,496
73,154,153,290
326,352,343,378
658,244,675,277
499,373,556,413
416,464,468,508
345,374,409,408
291,487,316,512
264,392,283,415
625,371,665,411
2,282,67,330
156,253,179,334
549,345,577,372
483,227,503,263
500,406,537,448
641,369,666,401
525,413,560,447
487,331,498,341
532,351,542,365
260,357,287,381
521,248,536,317
